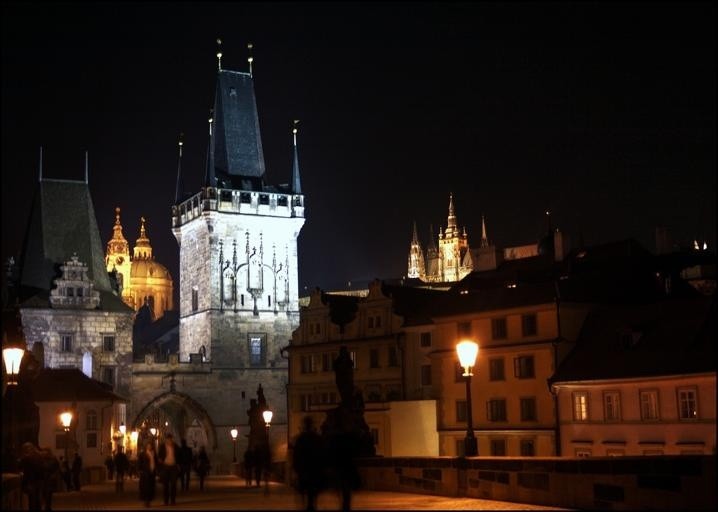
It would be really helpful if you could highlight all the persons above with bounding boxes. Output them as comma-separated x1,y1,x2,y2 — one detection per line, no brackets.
13,445,82,511
106,440,209,507
245,408,377,511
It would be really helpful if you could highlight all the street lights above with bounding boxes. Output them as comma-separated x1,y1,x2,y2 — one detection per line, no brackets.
2,340,28,474
59,411,73,492
149,425,157,450
230,425,238,463
263,408,273,495
455,340,479,457
118,421,125,455
